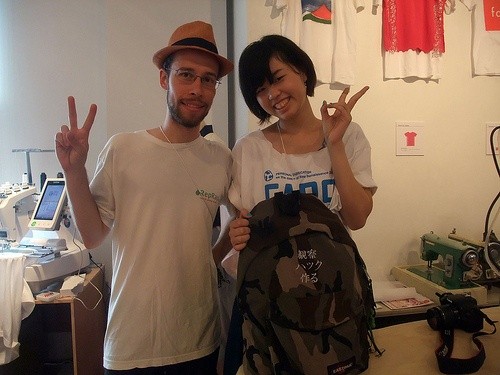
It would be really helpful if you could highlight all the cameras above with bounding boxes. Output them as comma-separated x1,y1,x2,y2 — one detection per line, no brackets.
427,292,483,333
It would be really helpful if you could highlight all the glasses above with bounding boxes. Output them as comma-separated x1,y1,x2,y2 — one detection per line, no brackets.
166,67,222,89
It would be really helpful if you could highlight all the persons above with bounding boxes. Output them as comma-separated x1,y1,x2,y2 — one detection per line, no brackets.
55,21,237,375
224,34,378,375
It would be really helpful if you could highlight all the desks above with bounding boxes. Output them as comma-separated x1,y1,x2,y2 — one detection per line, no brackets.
235,280,500,375
33,264,107,375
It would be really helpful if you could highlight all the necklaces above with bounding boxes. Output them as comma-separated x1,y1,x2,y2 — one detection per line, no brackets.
276,121,288,153
159,125,174,144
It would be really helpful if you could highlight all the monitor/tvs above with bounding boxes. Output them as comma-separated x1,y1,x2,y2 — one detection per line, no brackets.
29,178,67,231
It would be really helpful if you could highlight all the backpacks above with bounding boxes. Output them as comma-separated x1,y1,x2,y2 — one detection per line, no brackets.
224,189,375,375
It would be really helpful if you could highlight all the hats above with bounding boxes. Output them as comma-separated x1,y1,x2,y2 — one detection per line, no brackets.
152,20,234,78
